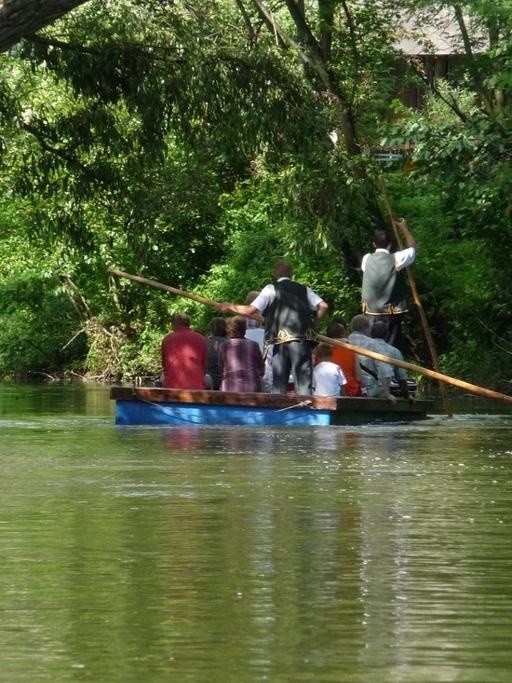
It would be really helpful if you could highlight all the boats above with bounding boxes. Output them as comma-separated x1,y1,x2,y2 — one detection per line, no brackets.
110,385,437,425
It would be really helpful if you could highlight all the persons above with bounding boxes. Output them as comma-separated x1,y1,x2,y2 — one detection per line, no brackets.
360,217,417,319
159,260,411,404
